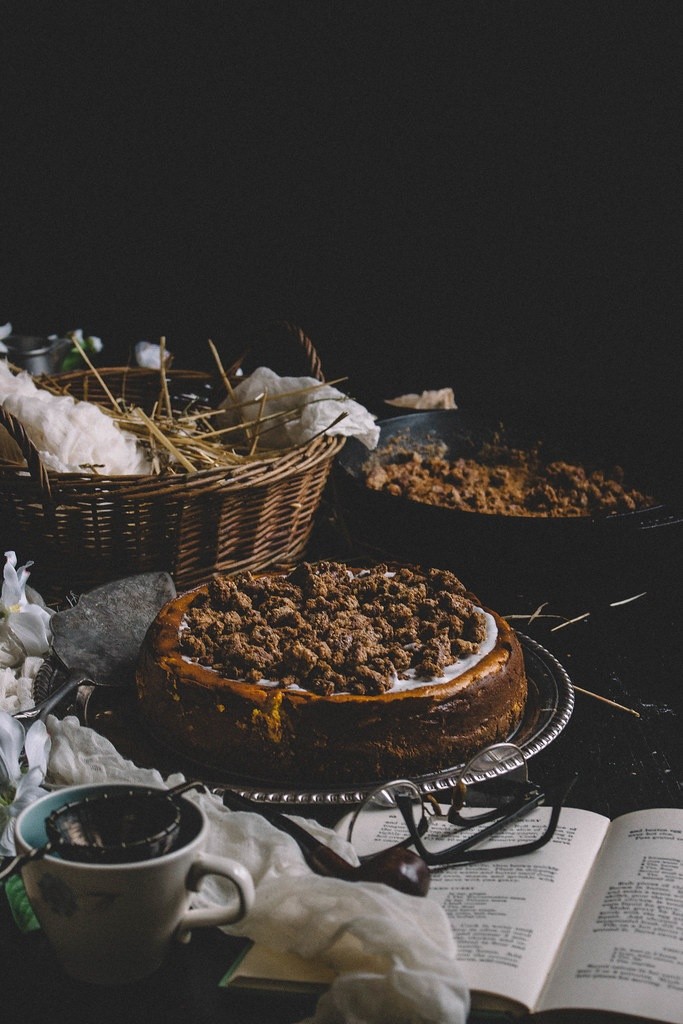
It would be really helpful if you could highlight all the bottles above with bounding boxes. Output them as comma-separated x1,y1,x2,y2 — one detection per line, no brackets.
0,335,57,377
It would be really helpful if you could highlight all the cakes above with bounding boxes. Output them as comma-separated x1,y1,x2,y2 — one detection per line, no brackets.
134,560,526,791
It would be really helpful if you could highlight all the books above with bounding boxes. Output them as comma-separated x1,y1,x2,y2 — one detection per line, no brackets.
219,796,683,1024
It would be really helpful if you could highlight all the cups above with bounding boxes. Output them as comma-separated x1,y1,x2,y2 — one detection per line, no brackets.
15,782,255,984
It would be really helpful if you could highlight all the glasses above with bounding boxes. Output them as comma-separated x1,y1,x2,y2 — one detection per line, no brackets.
346,741,583,867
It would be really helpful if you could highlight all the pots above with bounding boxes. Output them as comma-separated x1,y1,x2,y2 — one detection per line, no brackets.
339,408,683,610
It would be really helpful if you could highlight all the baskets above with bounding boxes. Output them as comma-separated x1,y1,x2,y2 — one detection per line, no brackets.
0,319,343,592
